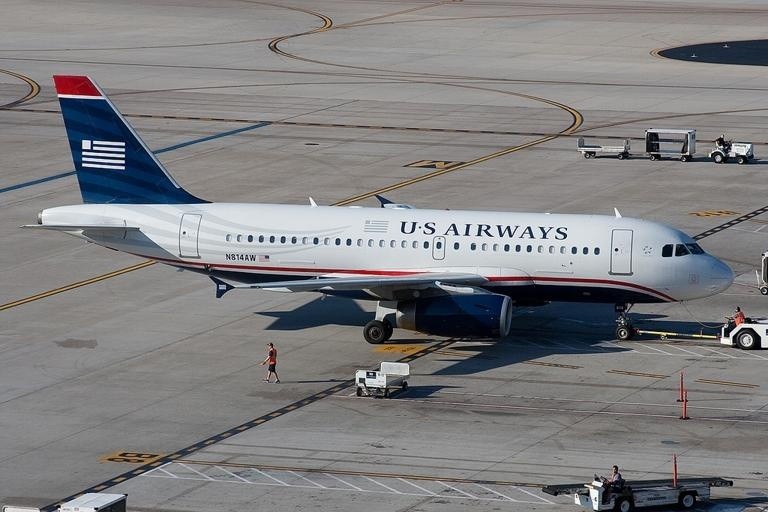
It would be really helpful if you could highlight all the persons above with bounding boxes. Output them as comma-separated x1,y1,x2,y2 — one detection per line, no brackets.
716,134,729,153
726,305,744,336
602,465,622,503
260,342,280,385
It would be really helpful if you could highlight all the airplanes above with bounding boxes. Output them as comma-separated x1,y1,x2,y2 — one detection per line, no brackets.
17,75,735,346
